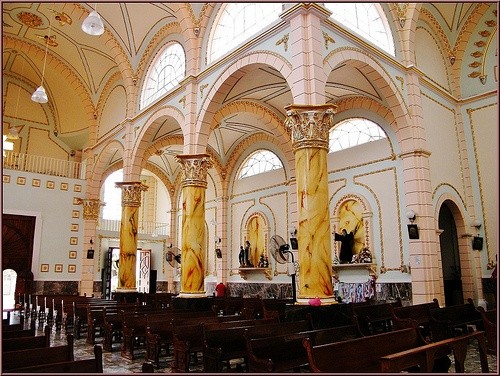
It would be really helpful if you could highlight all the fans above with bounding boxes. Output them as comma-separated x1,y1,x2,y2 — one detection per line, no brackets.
270,235,297,302
167,248,182,269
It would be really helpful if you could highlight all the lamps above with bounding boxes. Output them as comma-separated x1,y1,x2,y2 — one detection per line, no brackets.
474,220,481,230
8,59,25,140
406,209,417,224
82,3,104,37
31,4,55,103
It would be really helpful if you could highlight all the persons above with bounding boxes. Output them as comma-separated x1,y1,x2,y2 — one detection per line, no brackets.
334,229,355,263
239,241,253,267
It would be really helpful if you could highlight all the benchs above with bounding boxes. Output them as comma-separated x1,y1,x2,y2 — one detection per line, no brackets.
2,291,498,373
